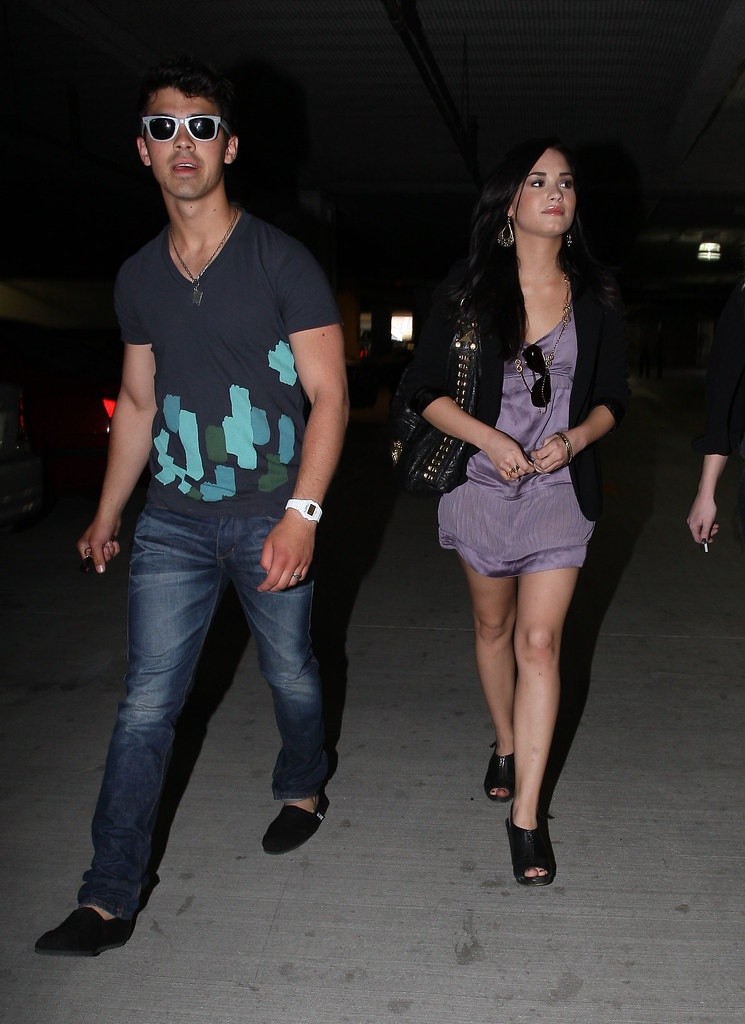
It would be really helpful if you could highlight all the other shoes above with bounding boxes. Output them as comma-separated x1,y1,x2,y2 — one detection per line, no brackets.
261,791,330,854
35,907,126,956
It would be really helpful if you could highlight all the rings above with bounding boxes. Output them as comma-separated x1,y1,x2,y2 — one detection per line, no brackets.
507,465,520,477
292,575,302,579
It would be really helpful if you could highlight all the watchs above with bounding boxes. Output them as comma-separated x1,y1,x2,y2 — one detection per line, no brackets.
285,499,323,524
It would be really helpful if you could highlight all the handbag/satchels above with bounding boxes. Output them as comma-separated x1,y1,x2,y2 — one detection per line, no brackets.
390,284,482,493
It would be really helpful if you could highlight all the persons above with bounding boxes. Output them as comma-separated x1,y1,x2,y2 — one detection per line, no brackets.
638,329,655,380
35,59,349,958
401,139,631,888
688,280,745,545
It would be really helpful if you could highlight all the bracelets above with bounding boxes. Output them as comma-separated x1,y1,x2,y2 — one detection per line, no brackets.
554,430,573,466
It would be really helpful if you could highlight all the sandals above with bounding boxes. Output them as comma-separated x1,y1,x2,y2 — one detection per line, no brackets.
506,802,554,886
483,741,516,803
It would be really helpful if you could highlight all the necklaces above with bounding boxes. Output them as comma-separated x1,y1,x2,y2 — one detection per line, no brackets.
169,206,238,307
515,272,571,413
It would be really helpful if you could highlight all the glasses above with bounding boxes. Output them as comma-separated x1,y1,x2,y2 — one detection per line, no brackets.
522,343,552,408
141,116,232,142
